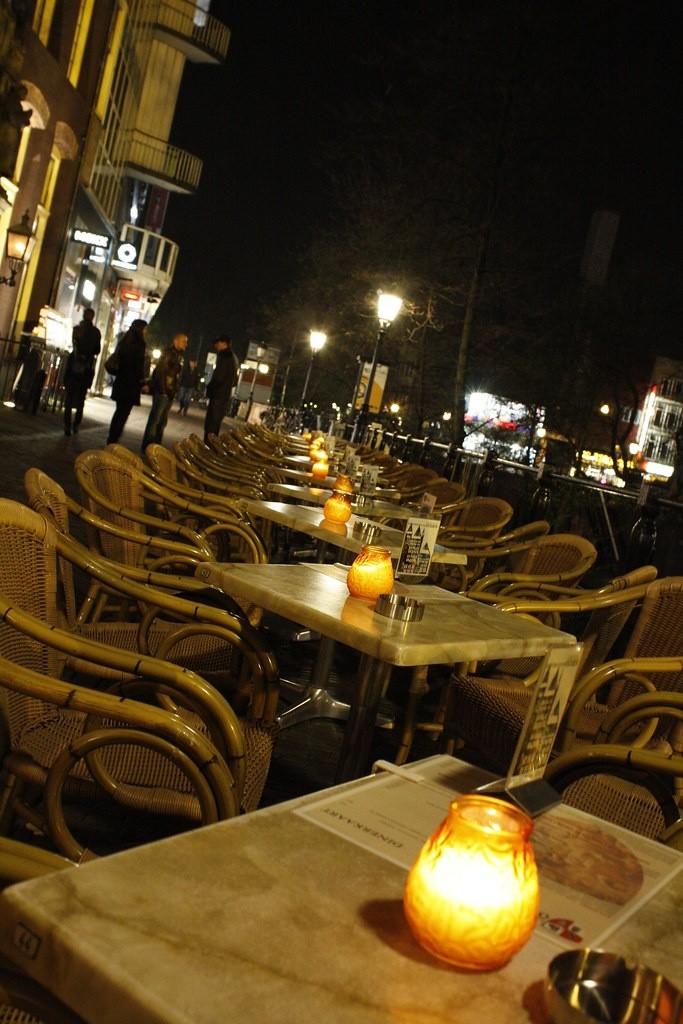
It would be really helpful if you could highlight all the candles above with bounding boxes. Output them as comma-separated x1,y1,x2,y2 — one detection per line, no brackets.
346,543,394,601
401,793,541,974
332,474,355,494
323,489,352,525
311,461,330,479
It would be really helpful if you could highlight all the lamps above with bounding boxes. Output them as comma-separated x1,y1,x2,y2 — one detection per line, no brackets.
0,209,38,288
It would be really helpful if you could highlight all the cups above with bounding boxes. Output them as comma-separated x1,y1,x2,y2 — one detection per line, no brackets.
323,492,351,524
347,545,394,599
332,475,352,493
311,462,329,480
403,795,539,971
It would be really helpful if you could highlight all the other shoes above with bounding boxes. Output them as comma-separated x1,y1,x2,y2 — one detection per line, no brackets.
65,426,72,435
73,427,79,433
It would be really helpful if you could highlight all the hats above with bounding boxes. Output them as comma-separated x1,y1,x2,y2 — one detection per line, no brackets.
212,334,232,346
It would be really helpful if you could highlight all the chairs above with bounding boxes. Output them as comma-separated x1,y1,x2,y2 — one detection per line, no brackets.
437,520,550,591
22,466,259,716
146,421,513,588
543,690,682,849
74,448,269,627
0,497,280,840
427,564,658,741
103,443,257,563
436,533,598,623
0,657,241,1024
442,575,683,760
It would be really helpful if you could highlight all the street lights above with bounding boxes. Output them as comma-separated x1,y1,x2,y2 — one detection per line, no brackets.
243,340,269,422
349,289,403,443
294,328,328,425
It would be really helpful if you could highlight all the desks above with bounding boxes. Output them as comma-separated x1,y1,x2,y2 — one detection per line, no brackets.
266,482,413,564
0,754,683,1024
277,468,402,560
194,560,577,784
237,497,468,735
283,455,389,486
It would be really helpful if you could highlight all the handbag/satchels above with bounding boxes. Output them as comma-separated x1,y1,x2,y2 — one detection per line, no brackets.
104,352,121,376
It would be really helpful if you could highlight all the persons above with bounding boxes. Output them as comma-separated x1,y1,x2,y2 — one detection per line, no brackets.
62,308,102,436
176,357,202,416
260,410,290,434
460,422,486,500
106,318,149,446
141,332,189,454
203,334,241,446
